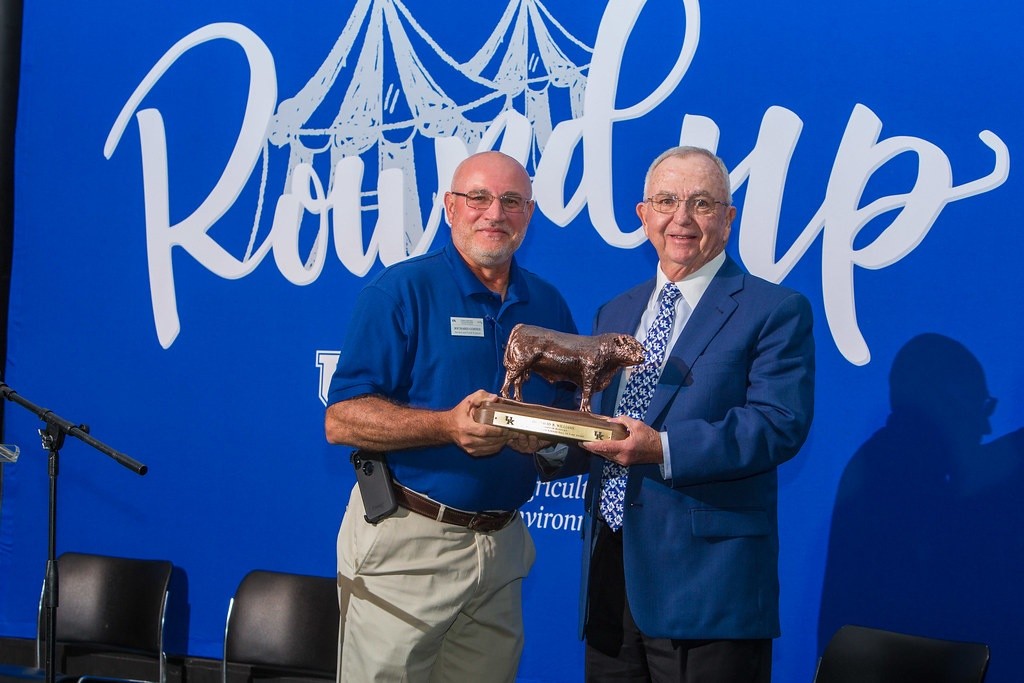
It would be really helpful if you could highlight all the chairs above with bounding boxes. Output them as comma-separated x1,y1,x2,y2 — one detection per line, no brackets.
813,625,990,683
37,553,173,683
223,570,341,683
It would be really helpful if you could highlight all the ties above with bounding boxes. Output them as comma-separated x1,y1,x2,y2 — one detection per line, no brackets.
597,282,682,533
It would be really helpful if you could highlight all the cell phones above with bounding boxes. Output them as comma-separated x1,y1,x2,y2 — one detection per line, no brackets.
352,448,398,524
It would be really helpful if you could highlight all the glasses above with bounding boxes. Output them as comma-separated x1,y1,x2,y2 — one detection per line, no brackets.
642,193,729,214
452,191,531,213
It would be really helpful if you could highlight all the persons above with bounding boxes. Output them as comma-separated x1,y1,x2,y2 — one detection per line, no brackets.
324,150,584,682
500,147,816,683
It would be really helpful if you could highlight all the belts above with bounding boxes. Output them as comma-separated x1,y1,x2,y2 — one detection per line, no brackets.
390,482,520,530
